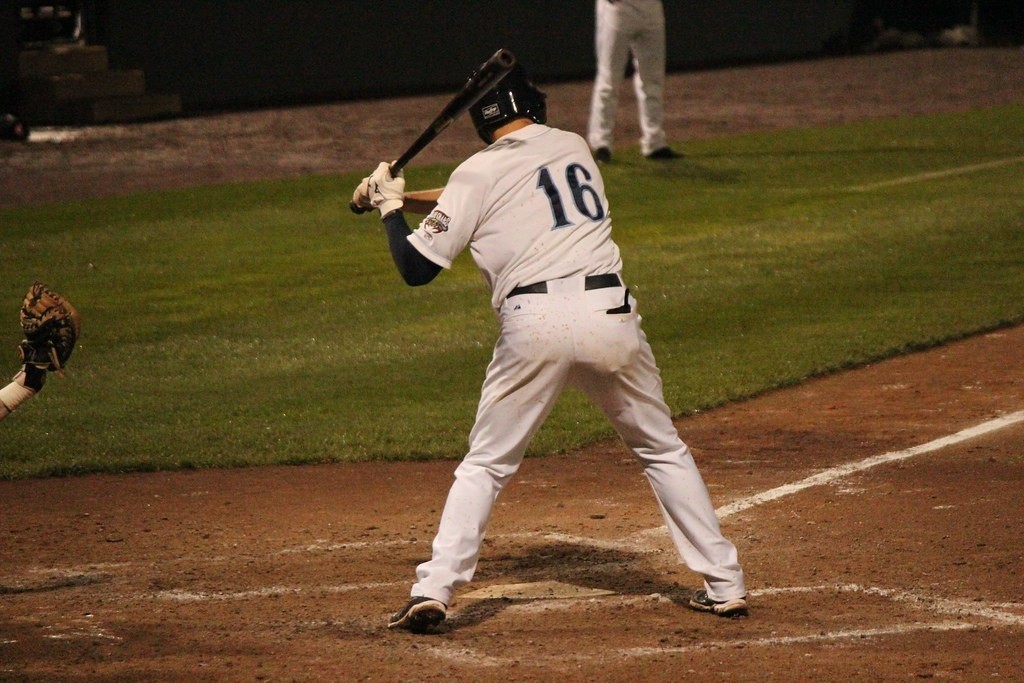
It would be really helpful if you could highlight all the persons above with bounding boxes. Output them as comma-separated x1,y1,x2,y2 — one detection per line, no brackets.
587,0,684,161
352,74,747,627
0,281,80,422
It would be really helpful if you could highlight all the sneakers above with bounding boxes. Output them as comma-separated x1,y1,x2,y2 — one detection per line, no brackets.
387,596,447,634
689,589,749,615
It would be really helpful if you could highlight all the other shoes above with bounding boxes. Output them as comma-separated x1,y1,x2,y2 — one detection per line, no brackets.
592,147,611,163
646,148,683,160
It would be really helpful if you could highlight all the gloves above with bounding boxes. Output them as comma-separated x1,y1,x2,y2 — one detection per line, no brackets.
367,160,405,219
353,177,380,212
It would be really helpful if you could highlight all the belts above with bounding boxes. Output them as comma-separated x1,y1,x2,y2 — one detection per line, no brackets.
506,272,621,298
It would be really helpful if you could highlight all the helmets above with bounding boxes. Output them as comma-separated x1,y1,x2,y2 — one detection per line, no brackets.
465,61,547,145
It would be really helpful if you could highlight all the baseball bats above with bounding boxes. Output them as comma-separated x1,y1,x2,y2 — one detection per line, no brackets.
348,48,516,215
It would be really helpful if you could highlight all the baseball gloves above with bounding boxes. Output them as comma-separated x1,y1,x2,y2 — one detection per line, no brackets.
17,280,79,378
